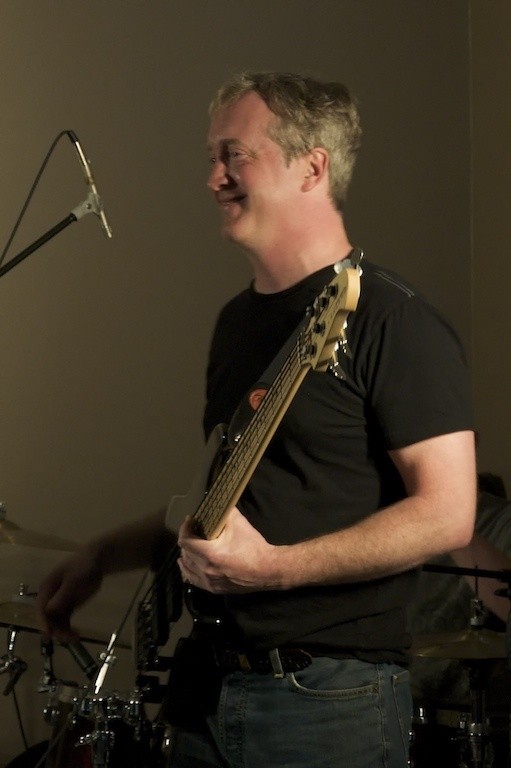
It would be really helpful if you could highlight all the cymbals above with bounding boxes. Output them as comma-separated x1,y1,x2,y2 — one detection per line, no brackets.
0,601,134,649
410,628,511,659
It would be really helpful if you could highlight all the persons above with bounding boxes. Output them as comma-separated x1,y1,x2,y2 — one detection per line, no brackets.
39,72,510,768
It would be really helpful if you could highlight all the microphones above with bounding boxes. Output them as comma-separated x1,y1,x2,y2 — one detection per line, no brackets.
67,131,112,238
68,640,100,680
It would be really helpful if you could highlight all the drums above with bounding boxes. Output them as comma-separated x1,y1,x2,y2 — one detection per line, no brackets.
410,708,496,768
42,683,162,768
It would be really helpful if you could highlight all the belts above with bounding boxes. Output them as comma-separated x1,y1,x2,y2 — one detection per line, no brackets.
174,637,313,674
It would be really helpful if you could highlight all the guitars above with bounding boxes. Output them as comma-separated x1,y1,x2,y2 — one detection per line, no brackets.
131,248,365,693
